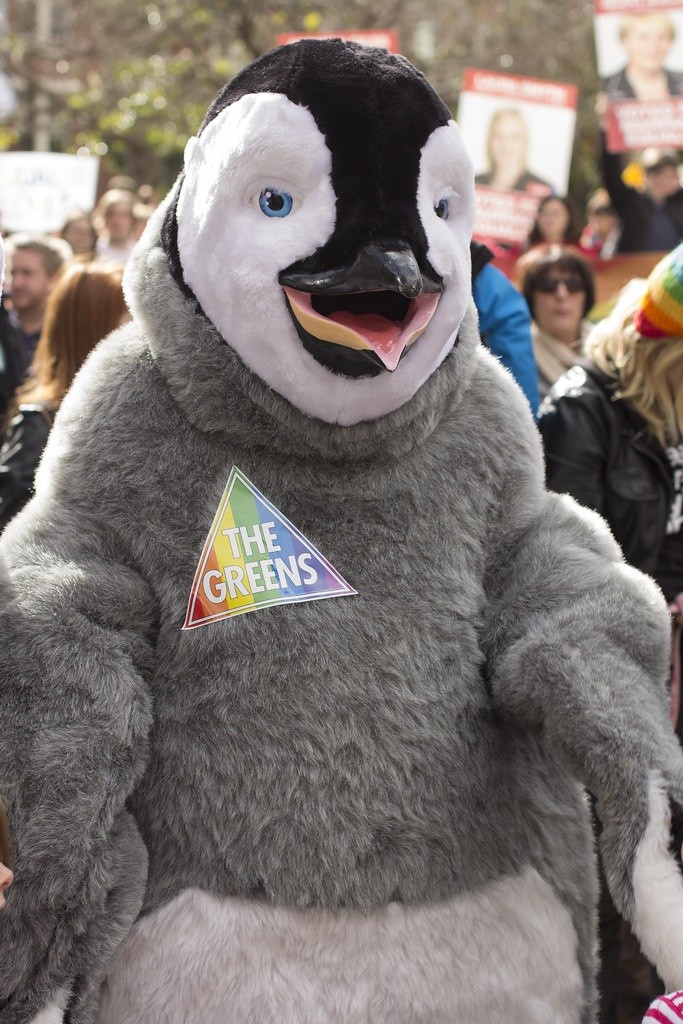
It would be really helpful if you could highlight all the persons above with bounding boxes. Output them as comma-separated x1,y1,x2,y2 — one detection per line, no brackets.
599,12,683,105
474,109,554,195
470,145,683,1022
0,185,158,537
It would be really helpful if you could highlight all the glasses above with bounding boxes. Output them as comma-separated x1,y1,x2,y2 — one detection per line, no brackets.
535,276,585,294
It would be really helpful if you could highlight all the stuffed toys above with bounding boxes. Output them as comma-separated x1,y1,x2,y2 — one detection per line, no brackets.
0,36,683,1022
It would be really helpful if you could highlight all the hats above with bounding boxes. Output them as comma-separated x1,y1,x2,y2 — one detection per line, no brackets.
634,240,683,338
